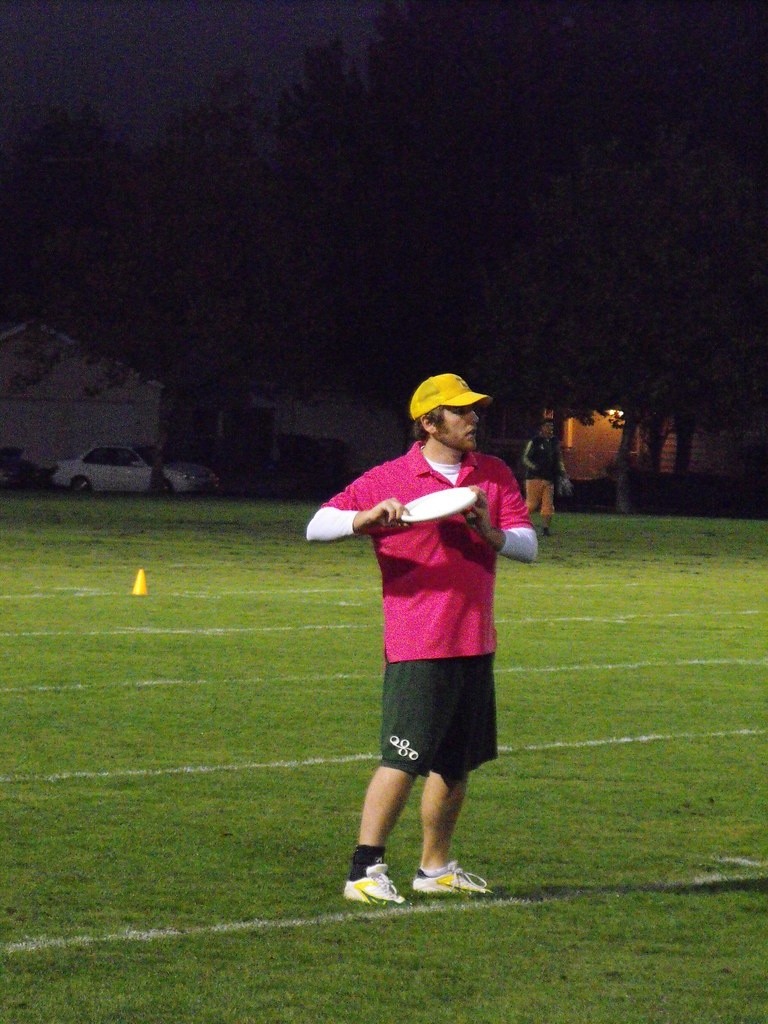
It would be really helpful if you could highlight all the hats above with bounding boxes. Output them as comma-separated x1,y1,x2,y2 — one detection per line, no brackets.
409,373,494,421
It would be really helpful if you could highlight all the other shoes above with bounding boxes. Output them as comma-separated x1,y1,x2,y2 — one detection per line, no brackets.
541,527,551,537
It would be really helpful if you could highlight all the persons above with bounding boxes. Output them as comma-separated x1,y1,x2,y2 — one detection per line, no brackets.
303,373,540,902
521,419,565,536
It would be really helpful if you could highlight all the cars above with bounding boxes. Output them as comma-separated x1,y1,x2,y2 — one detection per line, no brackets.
49,443,220,498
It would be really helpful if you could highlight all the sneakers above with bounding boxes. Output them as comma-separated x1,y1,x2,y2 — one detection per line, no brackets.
413,860,493,894
343,864,411,908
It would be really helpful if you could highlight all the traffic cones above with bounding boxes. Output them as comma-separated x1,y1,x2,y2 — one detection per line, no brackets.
129,567,151,597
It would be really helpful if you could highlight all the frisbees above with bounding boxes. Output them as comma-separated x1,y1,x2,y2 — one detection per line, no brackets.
398,486,479,523
